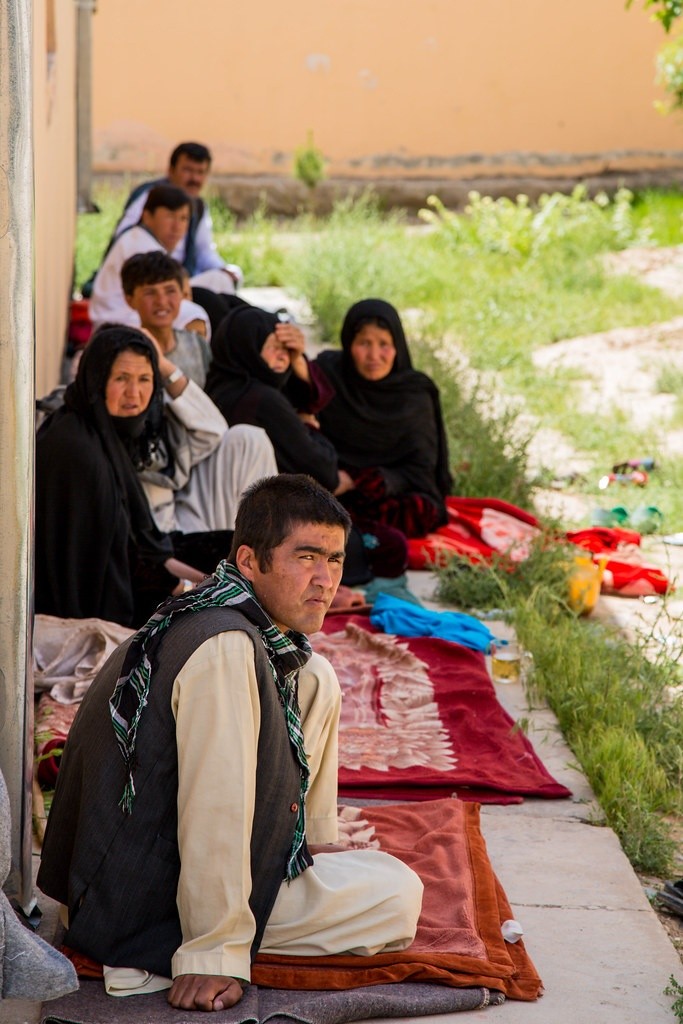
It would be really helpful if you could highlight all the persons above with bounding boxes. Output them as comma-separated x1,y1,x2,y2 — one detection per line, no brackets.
87,187,211,345
101,143,246,292
35,323,278,535
37,474,425,1010
202,309,341,497
121,252,213,390
35,321,235,631
309,298,454,539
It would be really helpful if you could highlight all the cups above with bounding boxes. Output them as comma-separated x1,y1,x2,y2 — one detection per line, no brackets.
490,638,534,684
